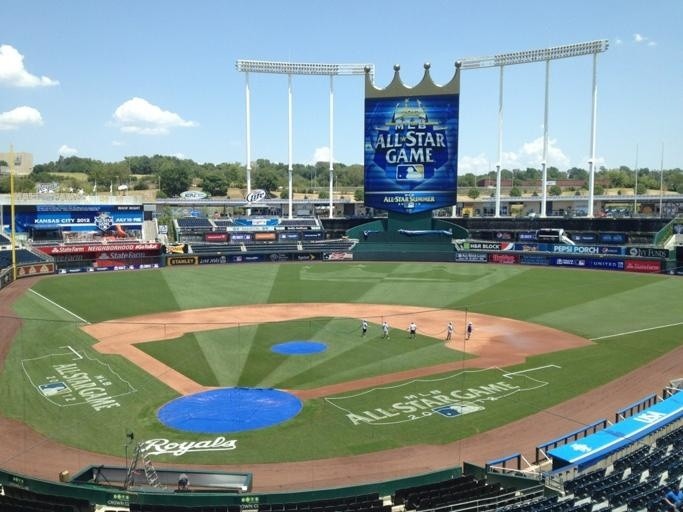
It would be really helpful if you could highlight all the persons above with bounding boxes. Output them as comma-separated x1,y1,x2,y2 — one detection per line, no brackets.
661,483,683,512
213,211,219,219
405,322,418,339
382,321,389,339
446,321,453,340
360,320,370,336
464,321,474,340
